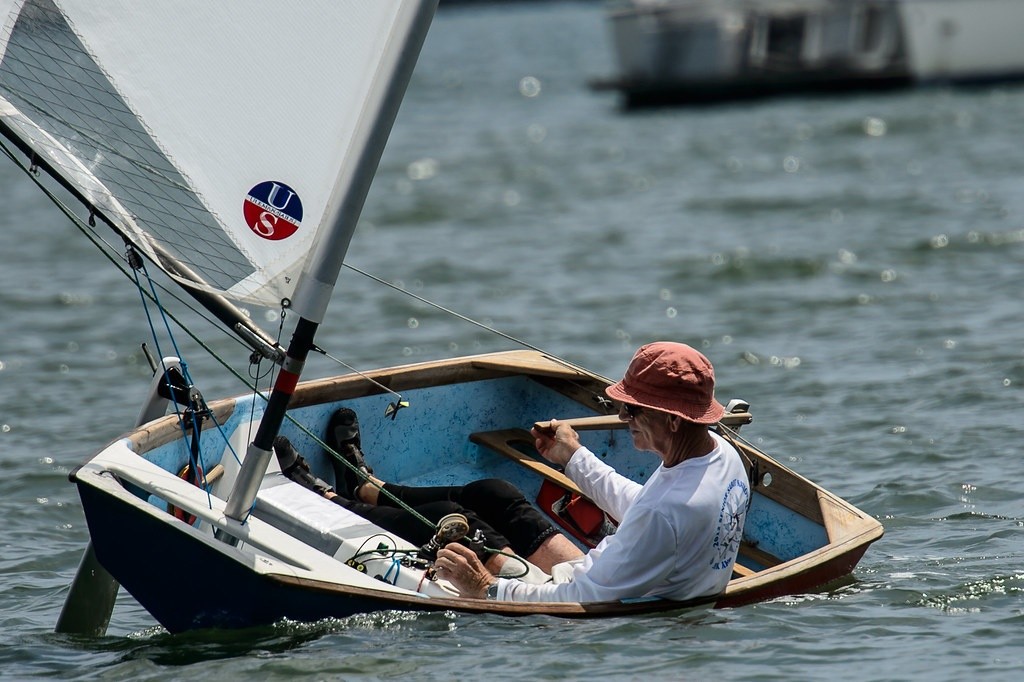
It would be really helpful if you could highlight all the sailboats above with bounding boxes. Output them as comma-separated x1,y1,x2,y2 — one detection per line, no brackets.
0,0,890,638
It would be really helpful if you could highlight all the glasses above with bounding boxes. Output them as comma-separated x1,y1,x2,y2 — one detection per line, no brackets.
622,404,644,419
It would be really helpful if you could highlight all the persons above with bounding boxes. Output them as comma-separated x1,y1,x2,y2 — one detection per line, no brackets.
273,341,751,602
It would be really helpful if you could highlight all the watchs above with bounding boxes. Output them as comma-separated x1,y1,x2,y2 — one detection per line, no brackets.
486,584,497,601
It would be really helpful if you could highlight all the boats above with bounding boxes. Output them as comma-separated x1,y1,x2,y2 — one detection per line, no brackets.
591,0,1023,112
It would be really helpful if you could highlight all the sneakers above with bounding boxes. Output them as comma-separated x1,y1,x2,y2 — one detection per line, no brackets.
326,408,374,500
276,436,333,496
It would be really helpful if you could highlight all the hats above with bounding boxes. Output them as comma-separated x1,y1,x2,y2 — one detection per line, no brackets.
604,342,726,424
